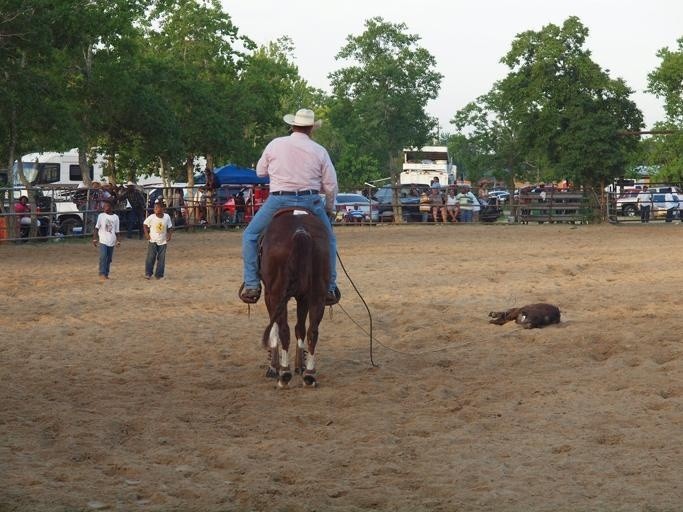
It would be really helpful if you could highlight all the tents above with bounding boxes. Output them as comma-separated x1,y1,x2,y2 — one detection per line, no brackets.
191,166,269,216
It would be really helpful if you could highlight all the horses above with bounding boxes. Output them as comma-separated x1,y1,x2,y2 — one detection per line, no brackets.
258,208,332,390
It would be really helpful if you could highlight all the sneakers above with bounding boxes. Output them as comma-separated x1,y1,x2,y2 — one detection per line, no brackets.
242,289,259,298
326,292,335,301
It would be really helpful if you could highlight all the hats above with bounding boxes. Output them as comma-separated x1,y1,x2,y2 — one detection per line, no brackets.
283,108,321,130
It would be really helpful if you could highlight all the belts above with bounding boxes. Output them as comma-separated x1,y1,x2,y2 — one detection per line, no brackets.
273,190,317,195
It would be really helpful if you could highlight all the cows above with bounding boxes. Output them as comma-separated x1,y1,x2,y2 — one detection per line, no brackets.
487,301,562,330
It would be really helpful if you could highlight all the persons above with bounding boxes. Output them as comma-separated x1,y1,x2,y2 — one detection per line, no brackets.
534,175,571,223
664,187,680,222
91,200,121,281
241,108,338,305
636,183,651,223
350,205,366,227
142,201,174,279
193,185,268,227
408,177,501,226
15,181,146,240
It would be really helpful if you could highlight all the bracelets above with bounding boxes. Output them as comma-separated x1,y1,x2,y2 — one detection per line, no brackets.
92,240,98,242
